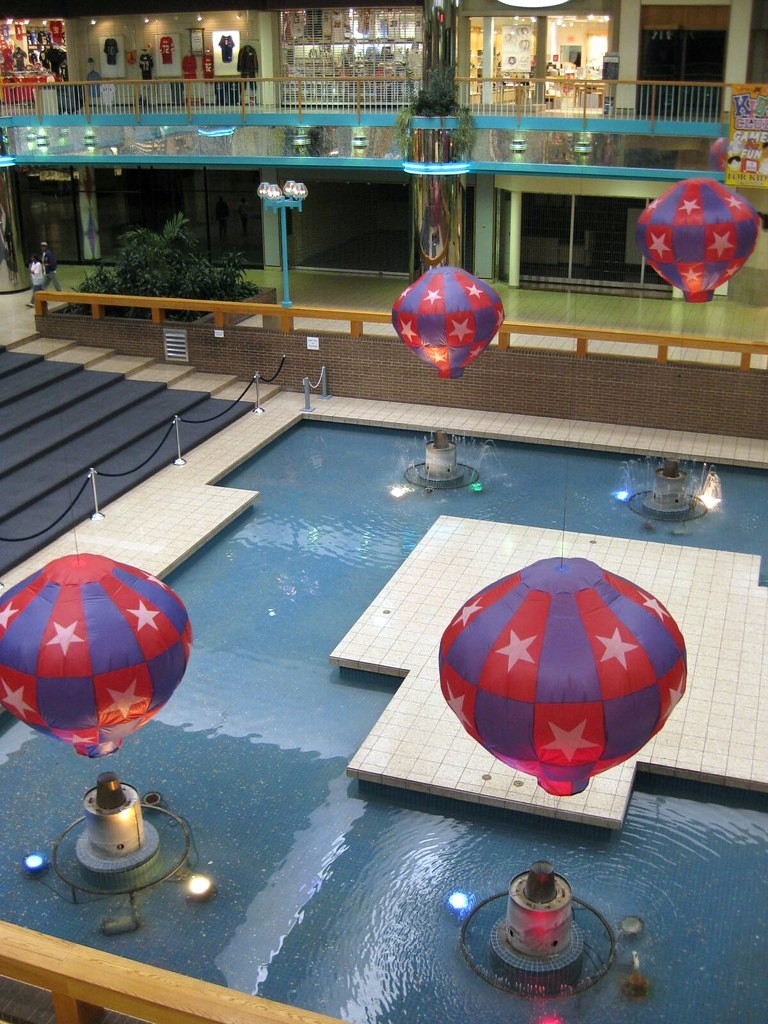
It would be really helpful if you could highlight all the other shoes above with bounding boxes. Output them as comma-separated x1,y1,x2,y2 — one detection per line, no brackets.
224,237,227,240
26,304,34,307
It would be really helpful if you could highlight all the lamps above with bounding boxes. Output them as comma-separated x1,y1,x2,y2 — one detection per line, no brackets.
83,135,96,147
354,136,367,149
575,142,593,155
510,140,526,153
36,136,49,146
293,135,311,148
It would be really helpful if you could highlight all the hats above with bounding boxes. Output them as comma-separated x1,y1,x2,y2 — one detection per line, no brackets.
25,64,34,70
34,62,41,69
40,242,47,245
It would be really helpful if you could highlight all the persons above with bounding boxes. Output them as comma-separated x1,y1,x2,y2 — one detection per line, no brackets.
40,240,62,291
238,197,249,236
25,254,46,308
215,195,229,241
